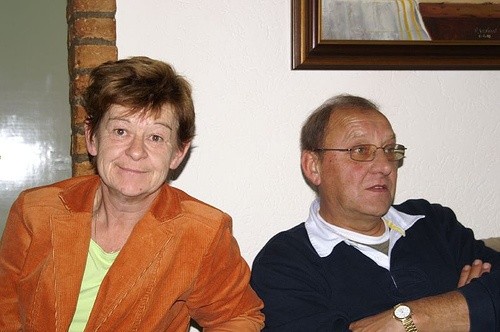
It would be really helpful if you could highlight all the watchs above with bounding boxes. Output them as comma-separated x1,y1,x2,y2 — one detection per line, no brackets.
393,303,417,331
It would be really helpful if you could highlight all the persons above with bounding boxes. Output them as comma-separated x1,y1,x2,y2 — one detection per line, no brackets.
0,56,266,332
249,94,500,332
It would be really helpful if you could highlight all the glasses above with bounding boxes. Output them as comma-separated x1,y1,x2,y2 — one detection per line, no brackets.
310,143,407,165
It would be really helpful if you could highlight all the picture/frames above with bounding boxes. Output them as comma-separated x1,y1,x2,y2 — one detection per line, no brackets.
291,0,500,71
